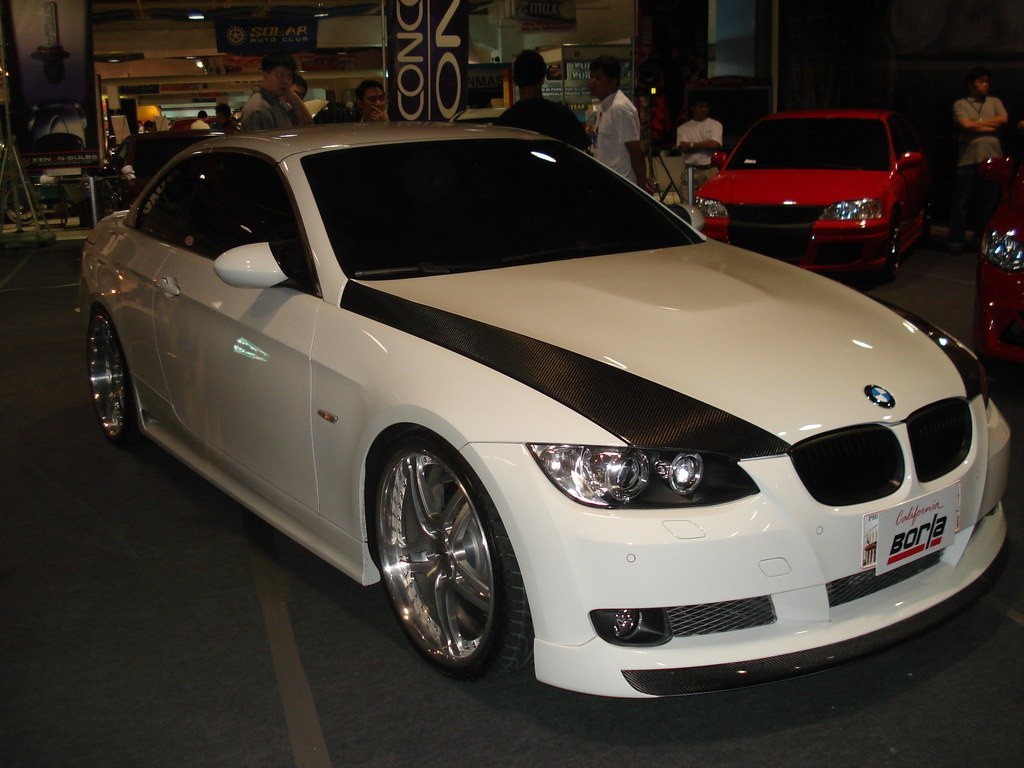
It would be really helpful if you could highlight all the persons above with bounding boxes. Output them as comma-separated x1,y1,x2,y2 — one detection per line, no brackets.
137,54,390,136
494,50,722,203
944,69,1008,255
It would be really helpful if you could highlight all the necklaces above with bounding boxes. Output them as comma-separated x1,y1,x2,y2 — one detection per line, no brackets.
964,96,984,123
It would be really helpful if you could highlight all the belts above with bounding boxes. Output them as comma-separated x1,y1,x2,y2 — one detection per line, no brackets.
685,163,713,170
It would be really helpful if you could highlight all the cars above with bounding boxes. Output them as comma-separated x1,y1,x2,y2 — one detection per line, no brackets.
77,122,1012,707
696,112,933,282
974,149,1024,368
108,130,214,216
